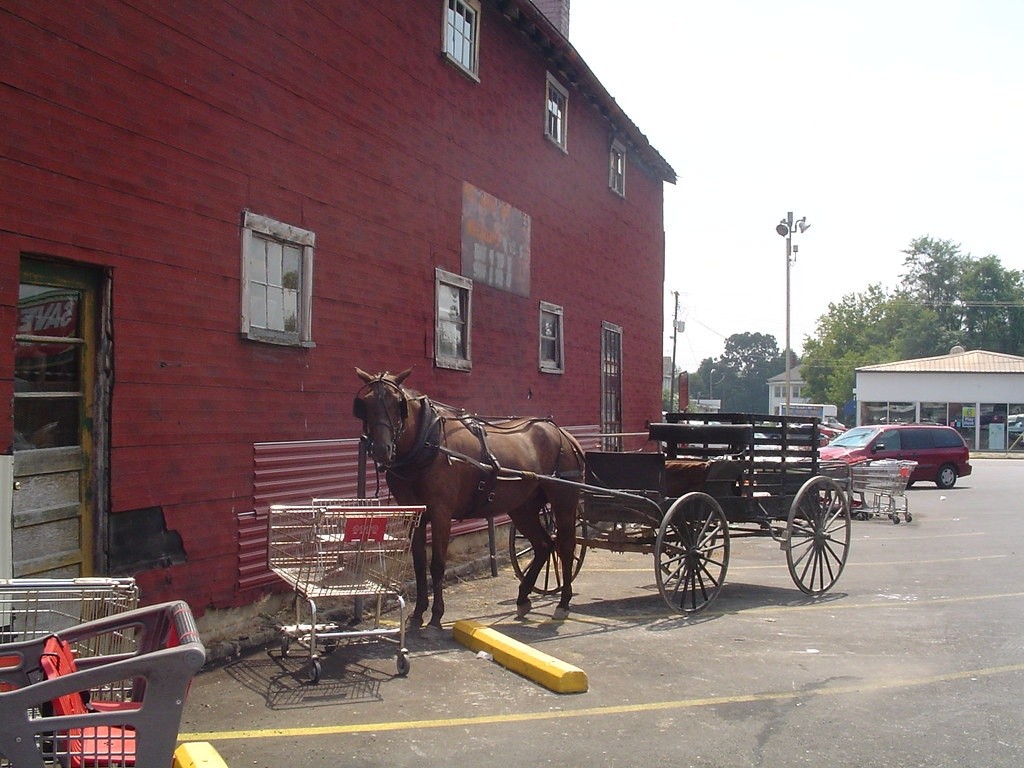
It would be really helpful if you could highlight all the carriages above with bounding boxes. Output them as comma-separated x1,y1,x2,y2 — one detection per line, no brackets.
350,364,853,641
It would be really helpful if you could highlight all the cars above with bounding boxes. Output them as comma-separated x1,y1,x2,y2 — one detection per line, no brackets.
1008,414,1024,448
660,410,849,462
799,424,843,438
769,429,829,446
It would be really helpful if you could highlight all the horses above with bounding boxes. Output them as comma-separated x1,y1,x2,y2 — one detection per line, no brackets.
352,363,586,640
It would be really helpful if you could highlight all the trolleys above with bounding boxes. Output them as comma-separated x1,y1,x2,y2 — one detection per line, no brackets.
262,501,427,687
853,457,919,524
0,599,204,768
0,578,140,704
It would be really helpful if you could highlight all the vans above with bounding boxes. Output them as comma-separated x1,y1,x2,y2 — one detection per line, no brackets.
818,425,974,489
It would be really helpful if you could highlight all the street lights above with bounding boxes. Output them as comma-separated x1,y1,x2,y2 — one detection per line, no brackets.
709,368,716,399
775,210,810,413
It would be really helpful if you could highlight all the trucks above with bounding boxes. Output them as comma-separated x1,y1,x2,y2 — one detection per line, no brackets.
779,403,845,428
